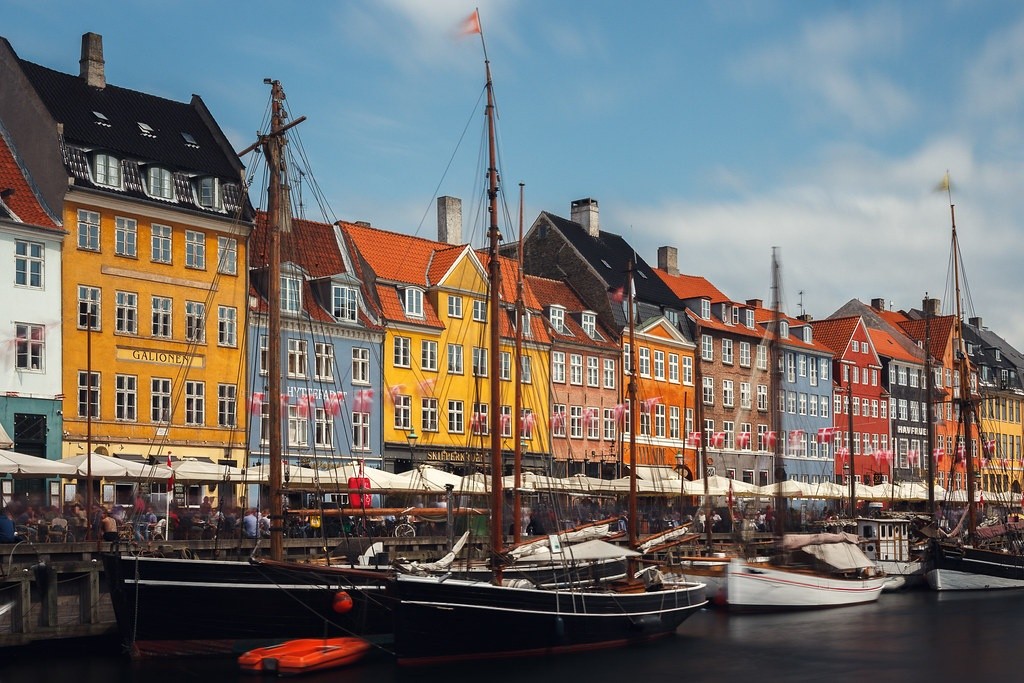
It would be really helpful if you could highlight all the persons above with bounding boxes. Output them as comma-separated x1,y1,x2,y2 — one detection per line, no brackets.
0,488,927,542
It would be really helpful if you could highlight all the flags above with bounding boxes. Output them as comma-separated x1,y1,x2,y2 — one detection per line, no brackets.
939,175,948,190
464,11,480,35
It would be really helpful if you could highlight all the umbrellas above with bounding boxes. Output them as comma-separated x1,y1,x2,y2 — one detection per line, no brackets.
0,450,77,474
165,461,268,481
246,460,349,484
56,450,173,478
326,465,1024,501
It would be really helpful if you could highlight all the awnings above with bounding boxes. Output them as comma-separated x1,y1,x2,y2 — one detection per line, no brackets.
627,467,689,482
0,422,14,449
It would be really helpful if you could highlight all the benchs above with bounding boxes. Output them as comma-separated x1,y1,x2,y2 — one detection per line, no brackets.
615,584,648,594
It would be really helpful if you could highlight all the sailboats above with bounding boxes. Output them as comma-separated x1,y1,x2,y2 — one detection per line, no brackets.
101,6,1024,672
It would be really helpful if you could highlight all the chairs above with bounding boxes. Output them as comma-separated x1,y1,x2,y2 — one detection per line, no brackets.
14,514,307,544
